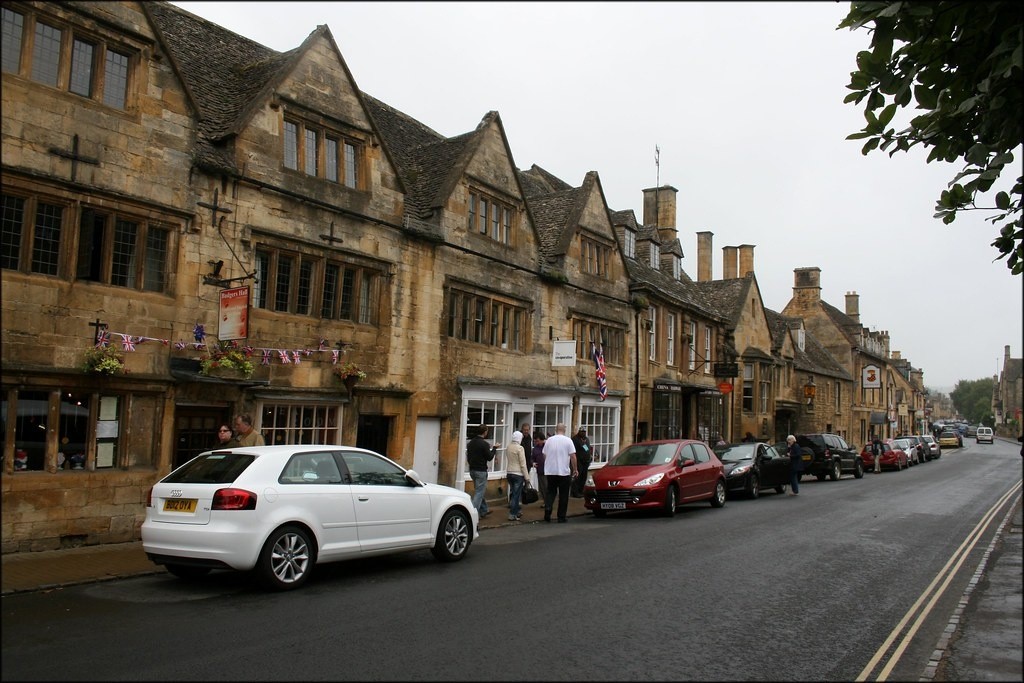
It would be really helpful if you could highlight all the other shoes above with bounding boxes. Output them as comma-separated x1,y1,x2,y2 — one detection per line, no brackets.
508,511,523,520
788,491,799,496
479,510,493,520
542,503,546,508
544,515,551,522
558,518,568,523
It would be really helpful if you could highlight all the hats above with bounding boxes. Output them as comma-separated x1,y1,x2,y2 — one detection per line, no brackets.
579,426,586,431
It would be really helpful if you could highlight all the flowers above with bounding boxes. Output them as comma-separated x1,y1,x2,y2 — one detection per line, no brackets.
334,363,365,385
197,338,255,381
83,341,132,380
70,453,86,462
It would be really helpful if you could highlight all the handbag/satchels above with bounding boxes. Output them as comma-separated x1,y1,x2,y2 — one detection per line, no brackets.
527,462,539,492
522,482,539,504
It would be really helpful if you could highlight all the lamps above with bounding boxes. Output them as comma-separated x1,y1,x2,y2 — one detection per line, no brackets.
802,378,816,404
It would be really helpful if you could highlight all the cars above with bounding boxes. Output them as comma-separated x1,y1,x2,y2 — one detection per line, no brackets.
710,443,792,498
139,444,479,589
922,436,941,459
938,432,960,448
931,420,977,447
895,439,919,466
582,440,726,517
860,439,908,472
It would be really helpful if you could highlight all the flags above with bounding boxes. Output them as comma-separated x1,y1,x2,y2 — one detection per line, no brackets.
592,344,608,402
98,331,343,365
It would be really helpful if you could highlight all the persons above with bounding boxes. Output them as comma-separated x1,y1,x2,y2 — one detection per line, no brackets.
872,435,885,474
786,435,802,496
467,424,501,521
716,434,727,446
506,422,591,523
213,414,264,448
896,427,939,442
741,431,756,442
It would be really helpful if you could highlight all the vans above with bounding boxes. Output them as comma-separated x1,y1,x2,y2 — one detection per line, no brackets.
975,427,994,444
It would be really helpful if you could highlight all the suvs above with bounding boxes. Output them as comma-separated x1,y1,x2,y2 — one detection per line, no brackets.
895,436,932,463
792,433,864,482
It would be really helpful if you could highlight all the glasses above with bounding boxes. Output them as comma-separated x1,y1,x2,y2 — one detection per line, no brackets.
219,430,231,433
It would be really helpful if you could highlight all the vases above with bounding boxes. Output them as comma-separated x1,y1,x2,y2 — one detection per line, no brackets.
209,361,249,380
73,461,84,470
344,373,361,388
